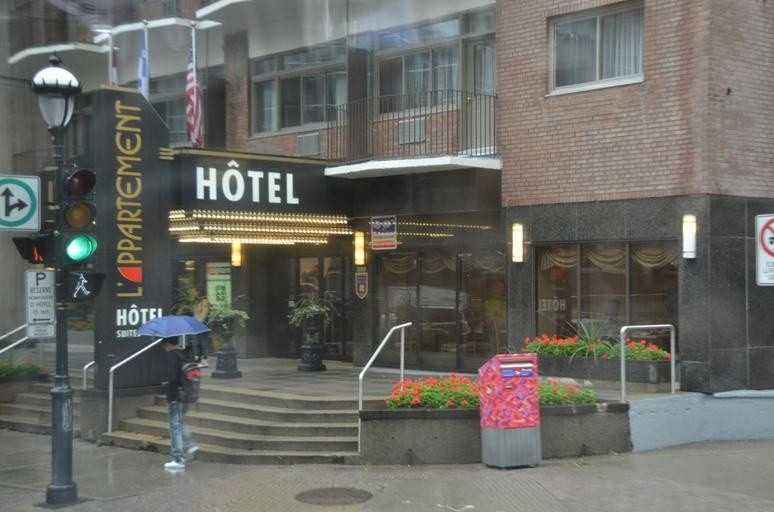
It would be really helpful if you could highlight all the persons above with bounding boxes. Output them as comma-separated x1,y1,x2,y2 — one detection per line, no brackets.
161,337,201,469
191,287,210,369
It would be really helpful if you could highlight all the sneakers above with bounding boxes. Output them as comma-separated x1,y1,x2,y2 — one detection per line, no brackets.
164,446,198,469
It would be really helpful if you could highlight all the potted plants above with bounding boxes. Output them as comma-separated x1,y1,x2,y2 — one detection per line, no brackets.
203,306,250,350
288,288,338,339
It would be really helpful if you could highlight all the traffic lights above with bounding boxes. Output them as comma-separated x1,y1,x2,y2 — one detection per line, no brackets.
11,236,46,264
62,168,104,302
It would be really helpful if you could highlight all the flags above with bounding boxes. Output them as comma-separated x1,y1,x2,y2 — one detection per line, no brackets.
136,26,150,101
184,29,205,149
108,39,117,87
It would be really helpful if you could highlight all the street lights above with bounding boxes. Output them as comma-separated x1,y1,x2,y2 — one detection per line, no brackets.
31,55,84,502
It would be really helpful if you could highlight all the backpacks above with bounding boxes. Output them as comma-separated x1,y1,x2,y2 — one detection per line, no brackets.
172,350,205,405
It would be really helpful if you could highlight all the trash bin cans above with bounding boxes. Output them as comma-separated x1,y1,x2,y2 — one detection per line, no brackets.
478,353,542,471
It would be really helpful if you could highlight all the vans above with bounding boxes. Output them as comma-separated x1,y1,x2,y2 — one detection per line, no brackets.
396,306,472,352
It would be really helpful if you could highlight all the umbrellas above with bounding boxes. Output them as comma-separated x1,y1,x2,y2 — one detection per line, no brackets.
134,313,213,339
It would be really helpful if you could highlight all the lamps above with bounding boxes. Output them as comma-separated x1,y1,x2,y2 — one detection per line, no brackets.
230,242,242,267
681,214,698,260
354,232,365,266
511,223,524,263
